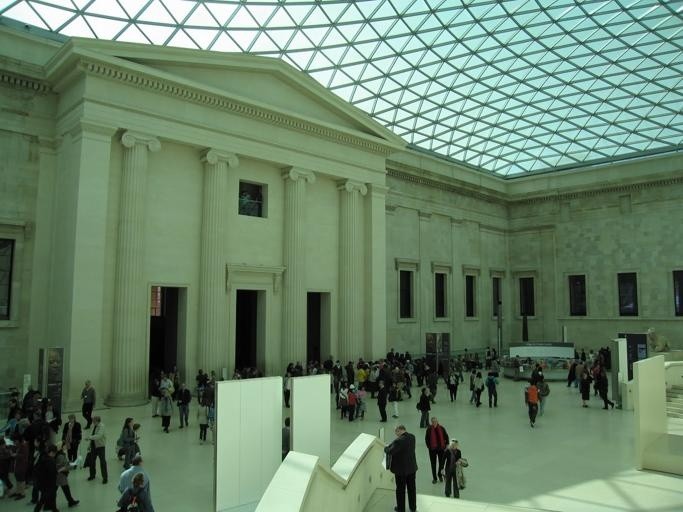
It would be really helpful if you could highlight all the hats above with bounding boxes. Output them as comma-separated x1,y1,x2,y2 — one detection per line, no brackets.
350,384,355,389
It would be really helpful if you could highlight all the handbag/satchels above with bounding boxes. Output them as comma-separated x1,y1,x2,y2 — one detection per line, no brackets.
83,453,92,467
417,403,420,410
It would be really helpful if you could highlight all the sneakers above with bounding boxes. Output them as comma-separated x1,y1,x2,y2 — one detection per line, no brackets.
432,476,443,484
87,476,95,480
152,414,160,417
179,422,189,428
164,428,169,433
102,480,108,484
7,492,79,511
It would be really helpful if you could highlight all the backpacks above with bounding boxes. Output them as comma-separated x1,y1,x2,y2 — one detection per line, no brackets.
126,486,144,512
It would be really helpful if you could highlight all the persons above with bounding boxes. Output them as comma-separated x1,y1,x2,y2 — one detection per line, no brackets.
425,417,449,485
0,377,152,512
38,349,61,381
383,424,418,511
438,439,463,497
282,415,290,461
153,346,614,446
